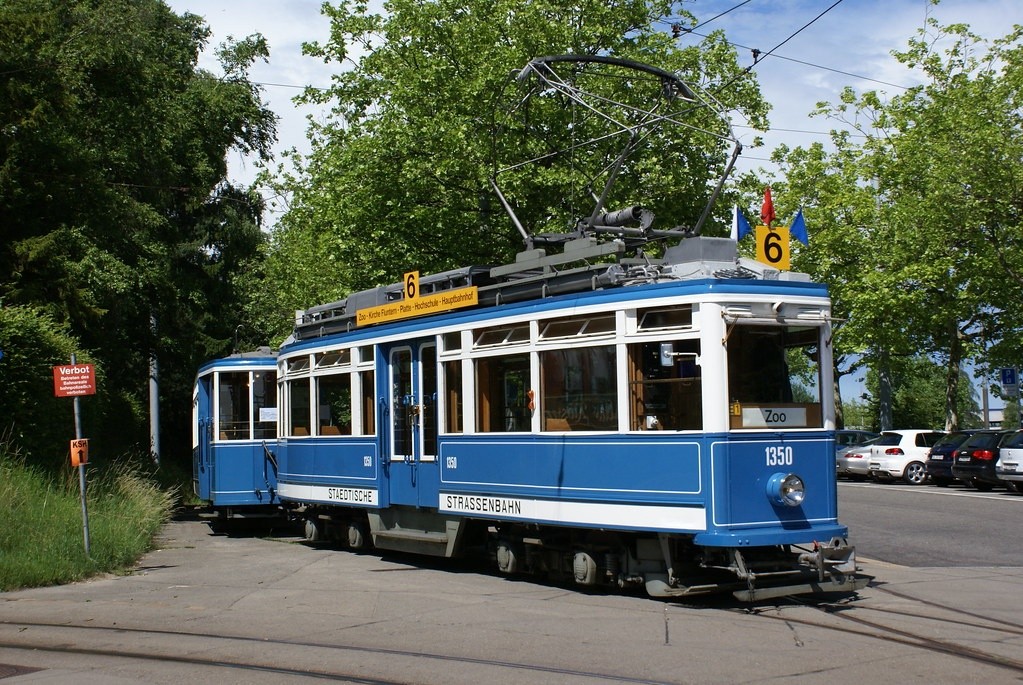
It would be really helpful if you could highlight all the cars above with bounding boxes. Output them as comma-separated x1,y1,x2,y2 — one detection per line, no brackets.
831,409,1023,497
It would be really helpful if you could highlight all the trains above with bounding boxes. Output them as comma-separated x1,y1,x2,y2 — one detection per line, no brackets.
185,204,872,607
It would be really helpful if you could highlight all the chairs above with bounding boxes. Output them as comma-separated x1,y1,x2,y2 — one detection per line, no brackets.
217,414,665,444
